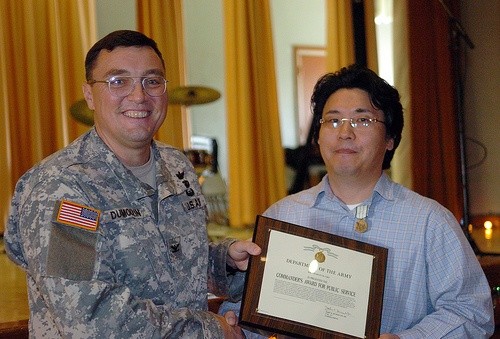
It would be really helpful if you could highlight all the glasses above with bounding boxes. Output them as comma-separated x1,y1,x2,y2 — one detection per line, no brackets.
86,76,169,96
319,112,387,129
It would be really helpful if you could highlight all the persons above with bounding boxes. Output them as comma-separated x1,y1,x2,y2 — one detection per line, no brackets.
216,63,495,339
3,31,262,339
